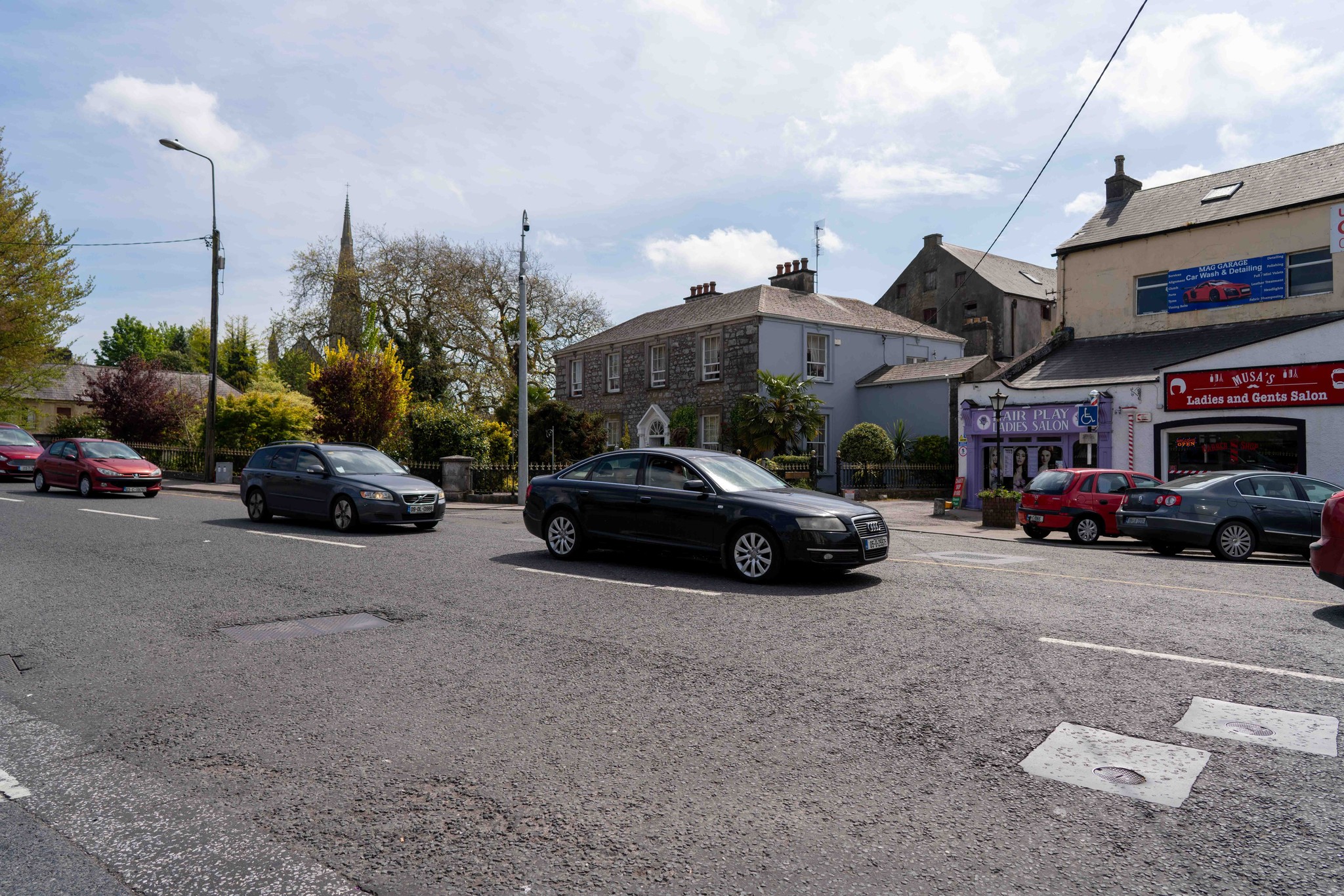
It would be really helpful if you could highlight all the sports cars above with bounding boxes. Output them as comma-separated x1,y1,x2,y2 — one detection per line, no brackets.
1183,279,1252,302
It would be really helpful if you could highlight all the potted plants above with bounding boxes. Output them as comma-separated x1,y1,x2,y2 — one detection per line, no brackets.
975,486,1022,528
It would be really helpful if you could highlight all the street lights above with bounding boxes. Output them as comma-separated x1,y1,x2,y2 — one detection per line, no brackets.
159,137,220,482
982,388,1017,528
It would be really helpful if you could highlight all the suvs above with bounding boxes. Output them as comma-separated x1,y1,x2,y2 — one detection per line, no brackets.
240,440,447,533
0,421,46,477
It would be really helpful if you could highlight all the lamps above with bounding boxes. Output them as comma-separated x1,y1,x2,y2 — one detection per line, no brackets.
1088,390,1099,429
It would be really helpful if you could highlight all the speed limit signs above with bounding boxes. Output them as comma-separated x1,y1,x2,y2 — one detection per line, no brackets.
959,446,967,456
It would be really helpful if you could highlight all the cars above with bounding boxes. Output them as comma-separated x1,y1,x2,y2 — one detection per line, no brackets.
1308,490,1343,589
33,437,163,499
523,447,890,584
1017,467,1165,544
1117,470,1343,562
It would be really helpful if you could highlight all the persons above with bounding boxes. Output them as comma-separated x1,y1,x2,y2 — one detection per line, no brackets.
661,464,686,490
1013,446,1028,491
1038,446,1053,474
989,446,1003,490
